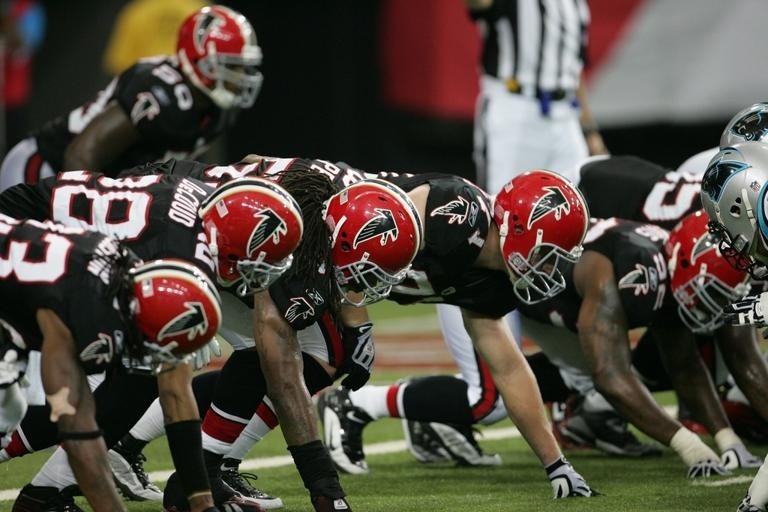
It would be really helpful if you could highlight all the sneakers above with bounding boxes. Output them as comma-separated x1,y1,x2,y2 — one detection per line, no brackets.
107,445,164,500
396,378,502,467
163,457,283,512
12,482,84,512
318,384,376,475
546,386,653,457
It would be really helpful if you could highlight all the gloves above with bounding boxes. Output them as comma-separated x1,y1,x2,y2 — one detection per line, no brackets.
545,457,603,498
189,338,221,371
716,428,761,469
335,321,375,390
670,427,732,478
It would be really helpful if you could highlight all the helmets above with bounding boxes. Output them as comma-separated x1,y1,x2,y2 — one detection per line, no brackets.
663,101,768,335
128,256,223,375
176,5,263,111
494,169,590,306
199,175,305,297
322,178,423,306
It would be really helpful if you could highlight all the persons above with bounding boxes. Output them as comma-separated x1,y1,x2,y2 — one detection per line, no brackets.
552,101,767,512
317,211,759,480
465,0,621,215
0,154,423,511
1,3,265,209
317,168,592,501
